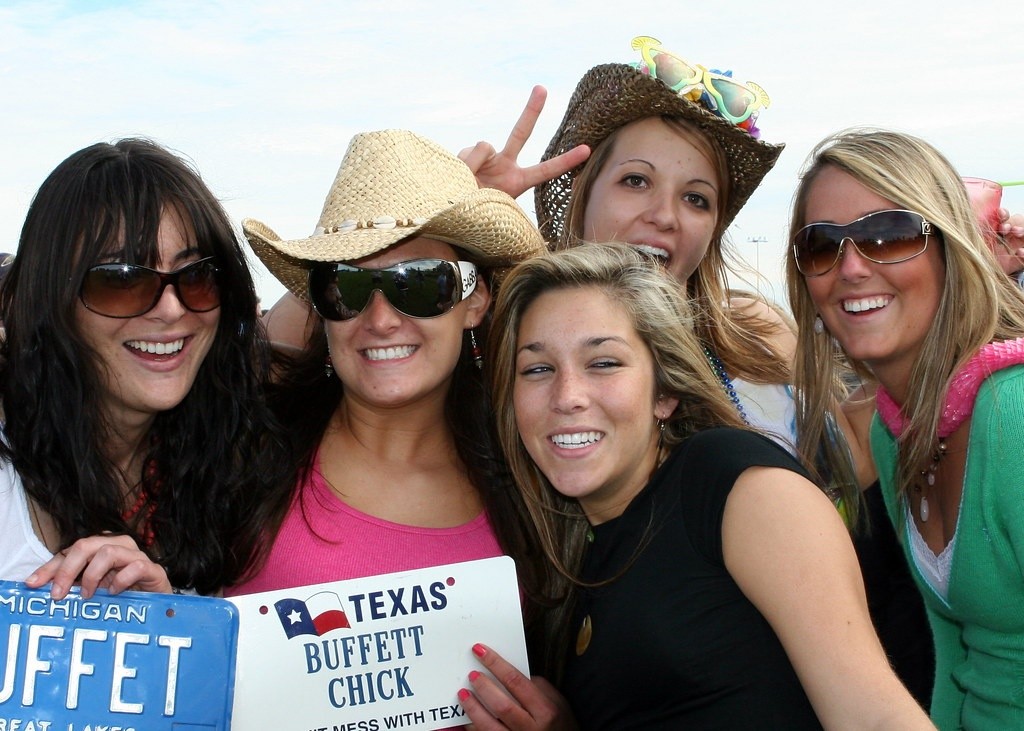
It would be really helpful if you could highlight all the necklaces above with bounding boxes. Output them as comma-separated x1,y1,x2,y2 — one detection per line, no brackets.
901,439,948,522
120,459,157,555
576,530,603,655
874,336,1024,448
705,343,749,425
699,342,729,399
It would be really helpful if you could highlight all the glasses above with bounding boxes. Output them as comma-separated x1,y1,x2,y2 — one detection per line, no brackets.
792,209,942,277
631,35,760,137
307,259,478,321
63,256,228,319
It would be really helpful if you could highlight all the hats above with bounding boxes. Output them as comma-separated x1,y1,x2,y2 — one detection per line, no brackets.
533,62,786,253
241,129,551,317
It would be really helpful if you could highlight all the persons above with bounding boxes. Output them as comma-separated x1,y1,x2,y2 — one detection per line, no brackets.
0,138,303,599
223,35,1024,731
